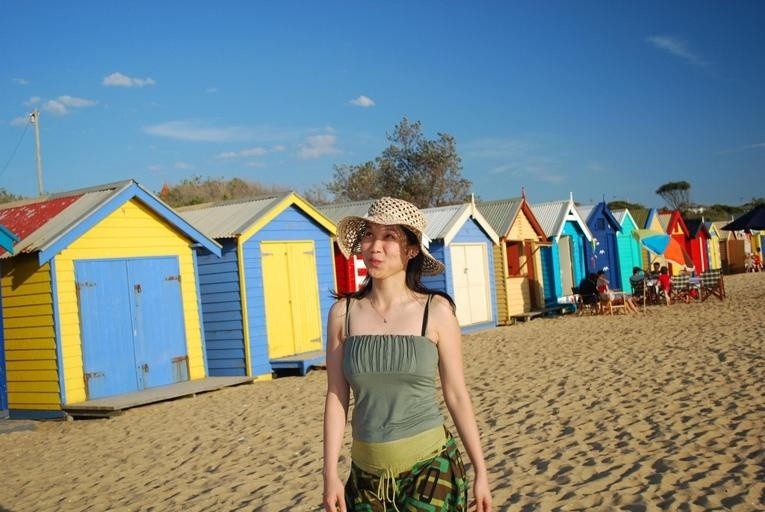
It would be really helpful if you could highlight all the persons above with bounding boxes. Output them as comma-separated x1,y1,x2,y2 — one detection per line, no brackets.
744,246,764,273
322,196,493,512
578,272,601,307
595,269,610,296
628,262,673,306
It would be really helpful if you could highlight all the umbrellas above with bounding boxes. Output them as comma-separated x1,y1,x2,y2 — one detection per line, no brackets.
719,203,765,232
640,233,696,272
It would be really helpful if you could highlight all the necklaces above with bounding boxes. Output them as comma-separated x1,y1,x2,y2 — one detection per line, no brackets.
367,291,418,322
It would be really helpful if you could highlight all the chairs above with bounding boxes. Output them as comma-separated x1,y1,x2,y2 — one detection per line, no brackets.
571,268,725,315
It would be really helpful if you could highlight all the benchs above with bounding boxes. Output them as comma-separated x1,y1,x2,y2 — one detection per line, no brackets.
509,301,573,324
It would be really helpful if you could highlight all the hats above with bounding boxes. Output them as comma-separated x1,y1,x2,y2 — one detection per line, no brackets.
334,193,447,279
